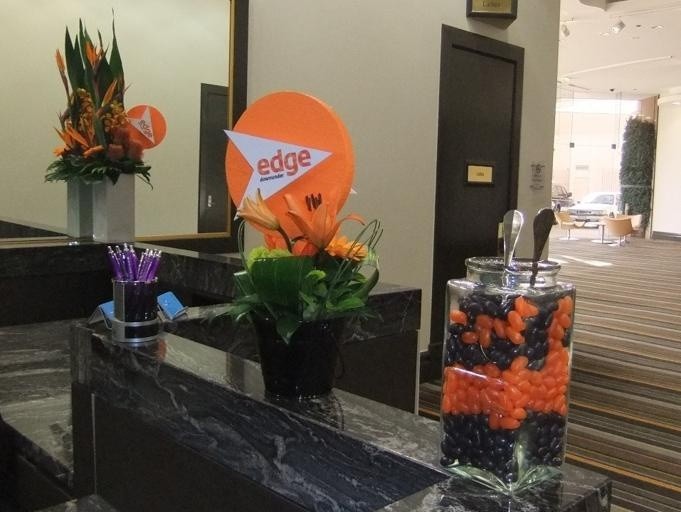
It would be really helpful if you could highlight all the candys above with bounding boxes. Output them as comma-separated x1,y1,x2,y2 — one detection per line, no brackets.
438,274,575,486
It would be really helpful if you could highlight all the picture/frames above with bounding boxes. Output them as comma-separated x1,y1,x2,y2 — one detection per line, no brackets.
466,1,518,20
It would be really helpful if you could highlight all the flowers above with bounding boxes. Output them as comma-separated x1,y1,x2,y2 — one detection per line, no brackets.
226,188,381,344
45,7,153,186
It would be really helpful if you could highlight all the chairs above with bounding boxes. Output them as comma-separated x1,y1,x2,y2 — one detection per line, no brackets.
555,207,642,249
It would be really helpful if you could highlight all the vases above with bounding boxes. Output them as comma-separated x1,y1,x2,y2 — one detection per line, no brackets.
248,307,349,400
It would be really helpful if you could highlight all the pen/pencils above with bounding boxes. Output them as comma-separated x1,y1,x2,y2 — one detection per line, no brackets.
108,243,163,281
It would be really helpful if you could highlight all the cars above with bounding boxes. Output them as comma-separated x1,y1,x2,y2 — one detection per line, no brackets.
563,192,627,228
551,182,576,225
